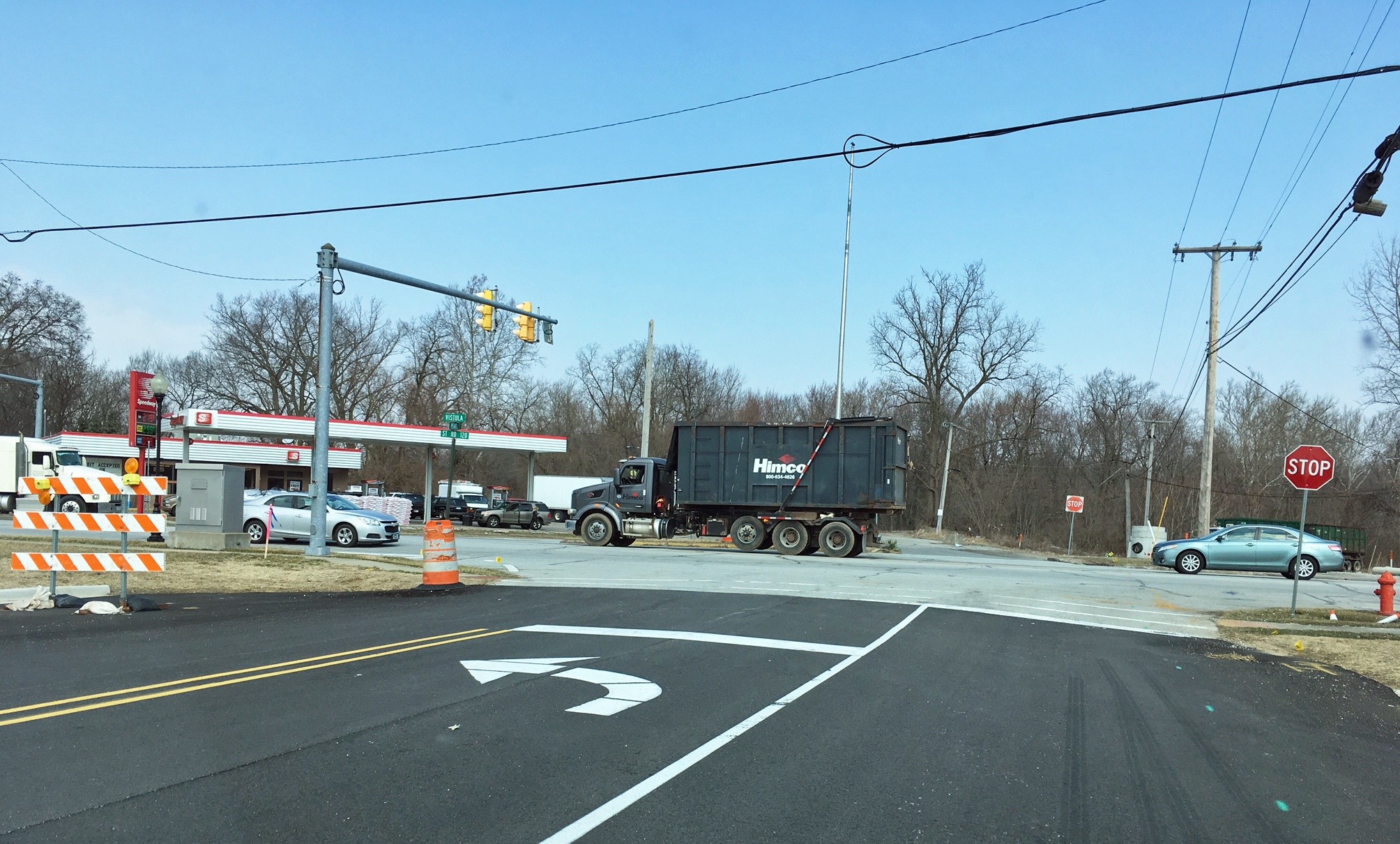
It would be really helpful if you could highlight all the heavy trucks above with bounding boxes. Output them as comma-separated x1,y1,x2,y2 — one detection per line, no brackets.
1191,517,1368,573
564,416,909,558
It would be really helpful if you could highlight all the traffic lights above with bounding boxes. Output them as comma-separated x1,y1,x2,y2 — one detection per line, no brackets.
476,291,493,333
514,302,534,342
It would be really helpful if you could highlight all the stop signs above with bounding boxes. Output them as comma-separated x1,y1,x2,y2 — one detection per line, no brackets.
1284,445,1336,491
1065,495,1084,513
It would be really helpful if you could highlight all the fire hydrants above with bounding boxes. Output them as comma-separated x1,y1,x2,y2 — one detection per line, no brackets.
1373,570,1396,615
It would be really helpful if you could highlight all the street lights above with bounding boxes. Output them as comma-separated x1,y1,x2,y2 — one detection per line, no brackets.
147,370,170,542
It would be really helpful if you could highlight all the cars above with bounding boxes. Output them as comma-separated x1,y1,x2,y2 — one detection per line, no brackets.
1152,524,1345,580
161,494,176,516
243,492,400,547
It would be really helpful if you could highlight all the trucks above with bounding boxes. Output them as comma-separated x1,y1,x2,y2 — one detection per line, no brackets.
438,480,488,521
532,475,613,523
0,431,122,514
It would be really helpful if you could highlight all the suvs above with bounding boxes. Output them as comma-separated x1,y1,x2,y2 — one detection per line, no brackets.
431,496,469,519
389,492,424,520
474,500,552,530
